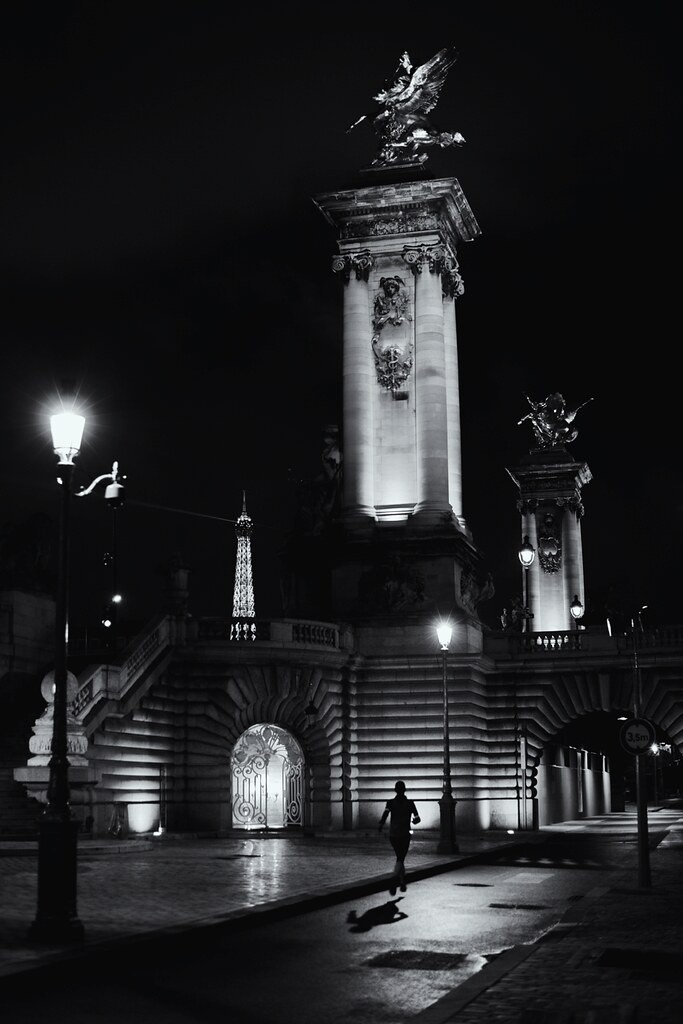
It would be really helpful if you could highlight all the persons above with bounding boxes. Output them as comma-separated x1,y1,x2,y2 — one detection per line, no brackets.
379,782,421,896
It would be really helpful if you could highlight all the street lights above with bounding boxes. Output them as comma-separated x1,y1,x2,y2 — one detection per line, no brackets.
26,410,87,966
436,620,461,854
605,616,654,888
